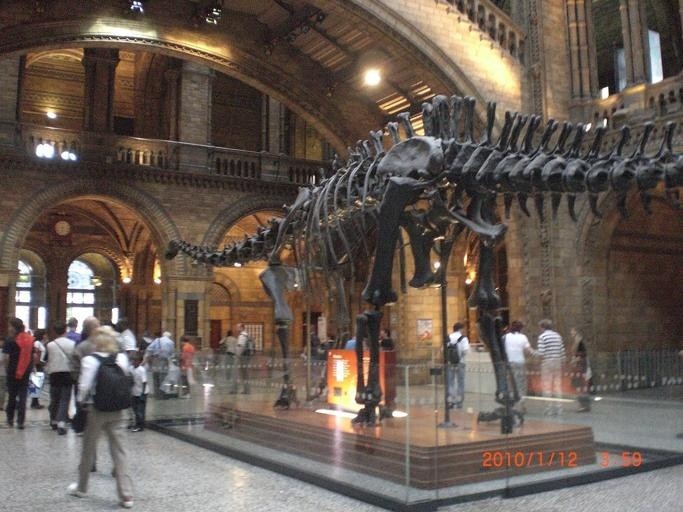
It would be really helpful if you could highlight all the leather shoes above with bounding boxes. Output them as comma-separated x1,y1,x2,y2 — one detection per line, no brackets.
229,391,237,394
240,391,250,394
0,404,83,436
131,426,145,432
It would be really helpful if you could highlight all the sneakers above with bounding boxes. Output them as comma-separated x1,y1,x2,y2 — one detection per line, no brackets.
542,406,592,416
447,402,453,408
457,402,462,408
118,496,135,508
68,482,88,498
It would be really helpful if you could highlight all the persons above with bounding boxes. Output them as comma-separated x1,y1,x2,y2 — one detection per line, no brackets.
70,317,124,476
340,328,396,352
218,329,238,382
531,319,566,416
440,322,469,408
500,319,535,413
237,322,253,395
0,317,195,434
568,326,593,412
66,333,134,508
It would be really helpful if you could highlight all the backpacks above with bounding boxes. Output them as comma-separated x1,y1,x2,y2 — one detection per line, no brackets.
238,333,256,356
91,352,131,411
448,333,465,365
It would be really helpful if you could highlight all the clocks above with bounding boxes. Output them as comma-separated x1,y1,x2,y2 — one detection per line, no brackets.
48,212,74,247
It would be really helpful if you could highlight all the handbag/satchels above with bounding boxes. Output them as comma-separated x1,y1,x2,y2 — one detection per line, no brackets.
70,360,78,381
216,343,225,355
151,356,169,374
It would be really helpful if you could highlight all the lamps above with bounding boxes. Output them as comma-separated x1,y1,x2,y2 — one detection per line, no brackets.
263,12,327,58
203,1,224,26
130,0,144,14
